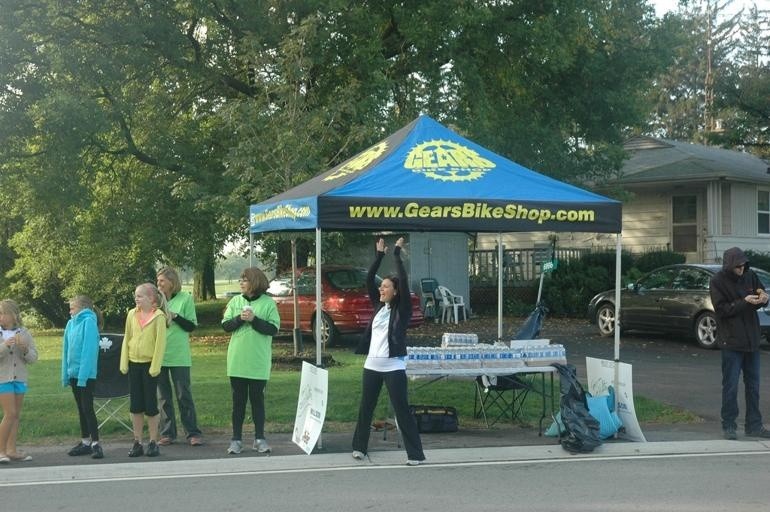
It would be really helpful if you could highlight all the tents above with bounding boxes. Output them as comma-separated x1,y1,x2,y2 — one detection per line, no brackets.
246,109,625,441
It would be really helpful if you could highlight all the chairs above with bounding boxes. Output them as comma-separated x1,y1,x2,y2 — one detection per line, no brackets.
439,285,469,326
94,332,146,437
419,278,441,319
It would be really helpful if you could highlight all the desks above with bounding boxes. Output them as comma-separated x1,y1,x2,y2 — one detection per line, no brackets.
381,367,561,439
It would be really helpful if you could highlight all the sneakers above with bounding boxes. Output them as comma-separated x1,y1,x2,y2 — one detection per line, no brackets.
128,439,144,457
745,423,770,438
91,443,103,458
252,438,272,453
227,439,244,454
158,437,176,445
67,440,91,456
146,440,160,456
723,423,737,439
407,459,424,465
353,450,364,460
191,436,202,445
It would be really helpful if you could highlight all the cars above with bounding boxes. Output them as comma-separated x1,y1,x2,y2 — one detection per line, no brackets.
588,264,770,349
265,264,424,347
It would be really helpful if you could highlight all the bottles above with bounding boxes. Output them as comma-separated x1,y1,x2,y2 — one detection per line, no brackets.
405,332,567,370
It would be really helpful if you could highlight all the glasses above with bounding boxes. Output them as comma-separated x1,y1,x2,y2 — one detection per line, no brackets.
238,279,251,283
736,264,744,268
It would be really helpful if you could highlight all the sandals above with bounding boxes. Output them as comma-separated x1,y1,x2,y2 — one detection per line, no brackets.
7,453,32,461
0,456,10,462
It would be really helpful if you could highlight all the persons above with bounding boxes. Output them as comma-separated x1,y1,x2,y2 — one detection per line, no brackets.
710,247,770,440
120,283,171,457
352,237,426,466
158,266,204,446
0,298,38,464
222,267,280,454
60,293,104,458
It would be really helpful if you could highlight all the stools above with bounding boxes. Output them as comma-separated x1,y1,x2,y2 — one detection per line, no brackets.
472,374,532,426
